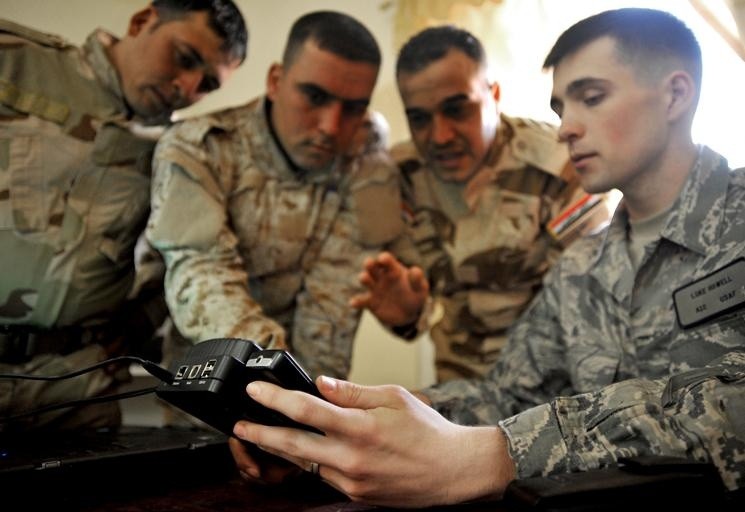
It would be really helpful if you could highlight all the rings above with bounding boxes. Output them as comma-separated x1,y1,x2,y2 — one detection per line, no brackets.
308,458,320,477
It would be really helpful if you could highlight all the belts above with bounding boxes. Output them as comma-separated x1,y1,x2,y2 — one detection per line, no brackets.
0,317,133,365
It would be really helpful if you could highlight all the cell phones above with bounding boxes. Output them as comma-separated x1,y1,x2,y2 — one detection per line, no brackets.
245,350,328,436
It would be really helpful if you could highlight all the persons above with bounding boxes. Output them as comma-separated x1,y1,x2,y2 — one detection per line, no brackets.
129,9,402,393
233,9,745,512
2,0,248,419
348,25,611,388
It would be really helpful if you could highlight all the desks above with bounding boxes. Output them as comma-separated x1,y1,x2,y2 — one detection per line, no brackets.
1,374,745,512
